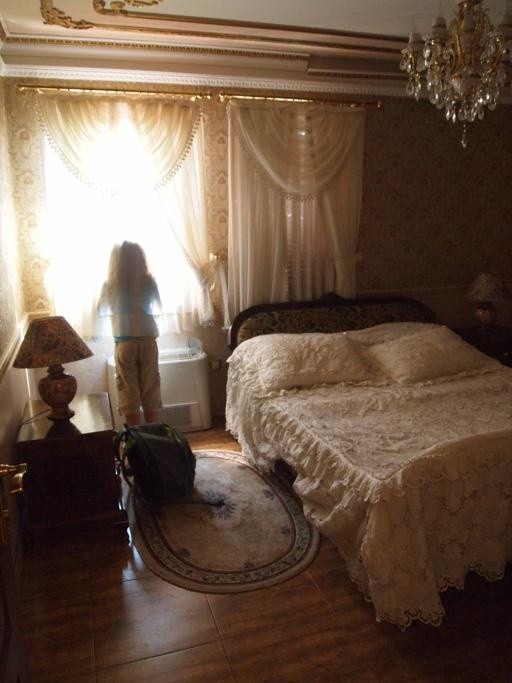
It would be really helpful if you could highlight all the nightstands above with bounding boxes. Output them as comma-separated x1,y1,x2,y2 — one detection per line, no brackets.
448,319,512,369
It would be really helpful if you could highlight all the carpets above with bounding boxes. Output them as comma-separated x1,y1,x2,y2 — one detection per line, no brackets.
123,449,320,595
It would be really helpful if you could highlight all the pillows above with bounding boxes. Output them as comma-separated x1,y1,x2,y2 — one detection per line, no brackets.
233,323,503,396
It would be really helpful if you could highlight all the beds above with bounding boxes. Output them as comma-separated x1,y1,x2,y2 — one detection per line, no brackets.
225,293,511,616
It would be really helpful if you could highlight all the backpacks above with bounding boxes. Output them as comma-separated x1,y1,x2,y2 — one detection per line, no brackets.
113,421,196,507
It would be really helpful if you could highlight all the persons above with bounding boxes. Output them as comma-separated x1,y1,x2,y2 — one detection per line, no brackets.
94,238,164,428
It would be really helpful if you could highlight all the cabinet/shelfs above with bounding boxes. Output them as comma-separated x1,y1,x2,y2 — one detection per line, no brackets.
13,393,129,547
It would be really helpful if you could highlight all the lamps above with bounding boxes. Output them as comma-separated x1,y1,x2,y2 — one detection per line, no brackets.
11,317,96,420
403,0,511,148
465,271,506,324
